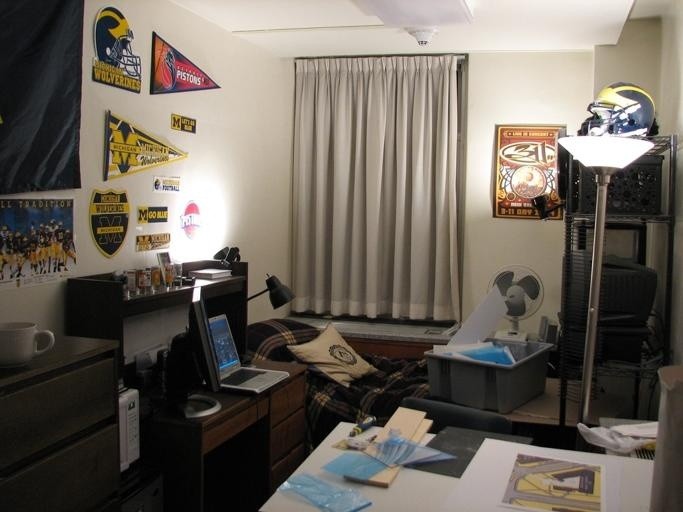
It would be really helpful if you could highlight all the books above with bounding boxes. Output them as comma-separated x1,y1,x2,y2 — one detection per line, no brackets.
188,268,233,279
344,406,434,486
497,453,607,512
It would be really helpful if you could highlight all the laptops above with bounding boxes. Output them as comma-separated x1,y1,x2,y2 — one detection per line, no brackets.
208,314,290,394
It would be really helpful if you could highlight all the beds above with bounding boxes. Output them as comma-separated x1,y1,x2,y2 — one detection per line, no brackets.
241,319,427,454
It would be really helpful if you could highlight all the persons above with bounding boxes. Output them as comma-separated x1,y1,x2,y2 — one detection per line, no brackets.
0,218,76,282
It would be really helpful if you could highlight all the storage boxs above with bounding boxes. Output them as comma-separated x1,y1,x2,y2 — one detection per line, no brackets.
422,337,555,413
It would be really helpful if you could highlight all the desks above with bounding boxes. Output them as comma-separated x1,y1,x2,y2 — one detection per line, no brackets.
257,421,660,512
137,351,308,512
423,373,660,429
67,258,248,402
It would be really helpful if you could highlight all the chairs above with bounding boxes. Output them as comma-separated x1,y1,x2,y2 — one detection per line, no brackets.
402,397,512,436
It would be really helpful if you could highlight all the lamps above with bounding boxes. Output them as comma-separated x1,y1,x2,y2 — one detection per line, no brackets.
247,274,296,310
558,134,655,448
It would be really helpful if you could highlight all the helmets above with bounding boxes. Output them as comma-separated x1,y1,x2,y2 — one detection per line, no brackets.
92,5,135,69
588,80,656,138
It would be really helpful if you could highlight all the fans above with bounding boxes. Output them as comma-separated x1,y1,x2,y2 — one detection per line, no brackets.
485,264,544,343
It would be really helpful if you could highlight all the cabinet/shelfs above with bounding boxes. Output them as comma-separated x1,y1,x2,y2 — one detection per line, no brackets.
0,335,121,512
556,132,678,449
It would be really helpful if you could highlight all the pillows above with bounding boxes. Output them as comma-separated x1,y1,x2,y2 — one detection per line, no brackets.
287,322,378,389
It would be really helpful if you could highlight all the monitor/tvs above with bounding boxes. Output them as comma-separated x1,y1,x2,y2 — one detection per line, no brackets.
560,250,658,326
170,286,223,419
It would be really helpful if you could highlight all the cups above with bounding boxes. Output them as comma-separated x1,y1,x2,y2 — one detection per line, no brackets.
173,263,182,287
1,322,55,370
151,266,160,289
